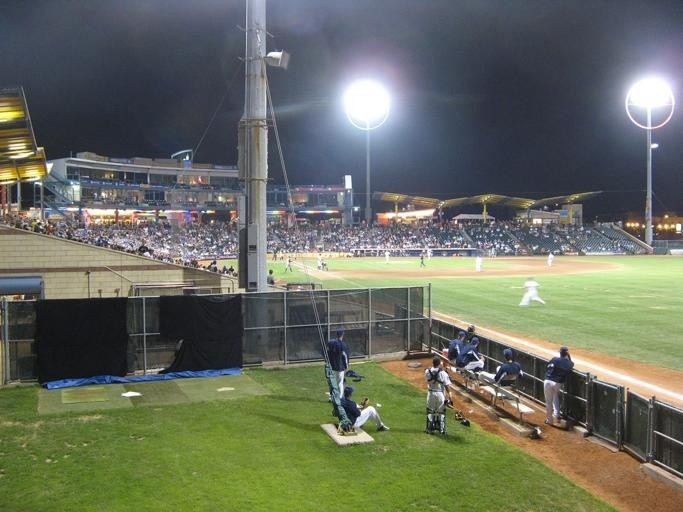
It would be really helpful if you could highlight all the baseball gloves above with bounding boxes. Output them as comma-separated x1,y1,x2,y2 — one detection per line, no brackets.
361,396,369,407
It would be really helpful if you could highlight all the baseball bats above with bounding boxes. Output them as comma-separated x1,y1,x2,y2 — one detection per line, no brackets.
510,286,523,289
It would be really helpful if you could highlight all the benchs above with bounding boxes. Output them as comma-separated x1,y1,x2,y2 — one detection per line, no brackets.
431,347,535,425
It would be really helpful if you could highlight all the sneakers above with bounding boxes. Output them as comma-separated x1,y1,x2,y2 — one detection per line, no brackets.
376,425,390,431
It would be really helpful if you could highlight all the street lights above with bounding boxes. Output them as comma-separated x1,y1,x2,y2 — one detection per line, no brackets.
630,78,668,246
346,81,387,226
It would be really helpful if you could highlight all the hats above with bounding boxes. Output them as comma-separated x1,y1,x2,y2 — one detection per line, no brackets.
458,331,466,337
343,386,354,394
503,349,512,358
335,327,344,337
558,346,568,353
470,337,478,346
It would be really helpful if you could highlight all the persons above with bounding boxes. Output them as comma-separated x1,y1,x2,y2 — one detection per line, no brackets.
423,356,453,436
476,256,482,273
542,346,574,426
323,329,349,403
516,278,545,308
455,337,485,382
447,331,466,375
548,252,554,266
338,385,390,431
0,213,239,277
317,254,328,271
267,270,279,285
267,215,624,261
493,349,523,384
384,250,391,264
285,256,293,272
419,254,426,267
